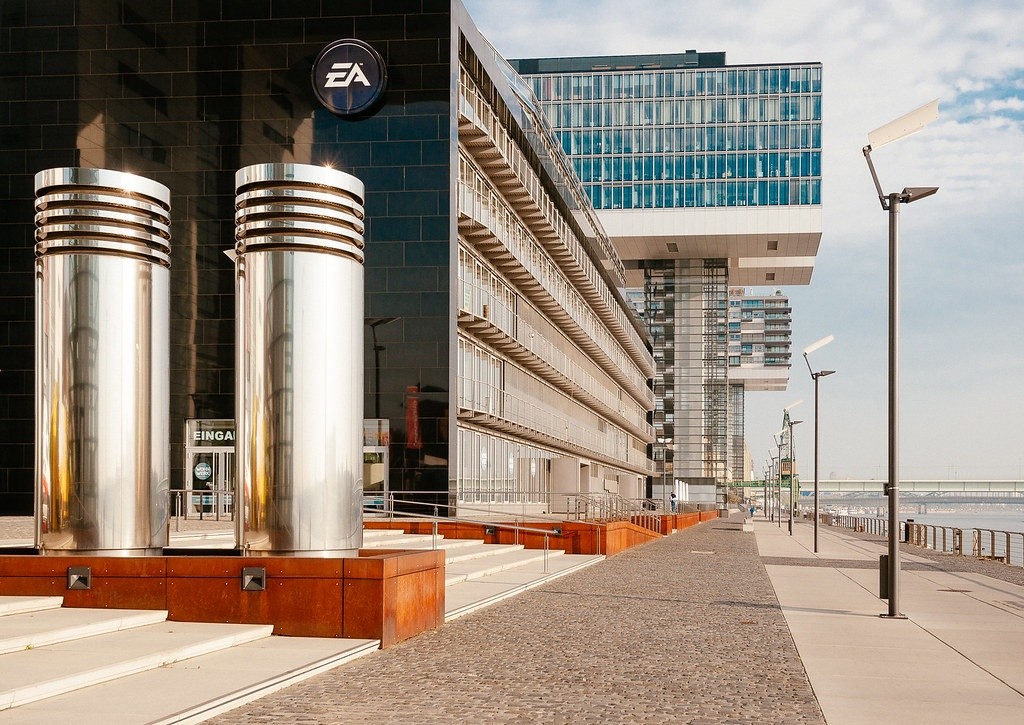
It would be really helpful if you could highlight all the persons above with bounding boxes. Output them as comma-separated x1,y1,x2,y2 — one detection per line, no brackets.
669,492,676,512
750,507,754,517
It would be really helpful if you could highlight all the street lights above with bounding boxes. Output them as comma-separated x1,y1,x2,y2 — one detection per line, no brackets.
766,457,774,521
801,334,837,554
858,97,943,620
768,446,779,522
773,428,789,528
366,316,404,419
761,466,770,516
783,401,804,535
